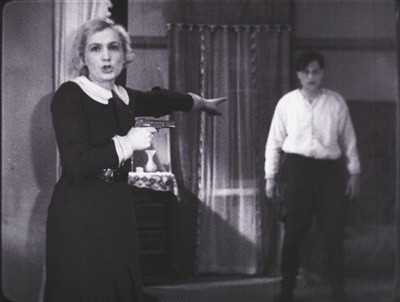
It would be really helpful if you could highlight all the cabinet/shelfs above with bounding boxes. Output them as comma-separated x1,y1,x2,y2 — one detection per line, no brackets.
129,170,175,286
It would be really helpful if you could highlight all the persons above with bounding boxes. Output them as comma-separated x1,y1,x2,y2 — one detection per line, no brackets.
264,52,364,301
41,17,228,301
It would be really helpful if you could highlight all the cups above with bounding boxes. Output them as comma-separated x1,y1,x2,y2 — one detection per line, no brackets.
136,166,144,172
161,165,169,172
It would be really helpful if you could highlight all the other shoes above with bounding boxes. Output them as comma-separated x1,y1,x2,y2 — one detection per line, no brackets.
332,285,348,302
273,288,293,302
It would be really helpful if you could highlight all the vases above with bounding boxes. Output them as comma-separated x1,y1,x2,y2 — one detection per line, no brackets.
146,150,157,171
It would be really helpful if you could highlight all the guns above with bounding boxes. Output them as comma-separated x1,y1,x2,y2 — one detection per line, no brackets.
135,117,175,134
